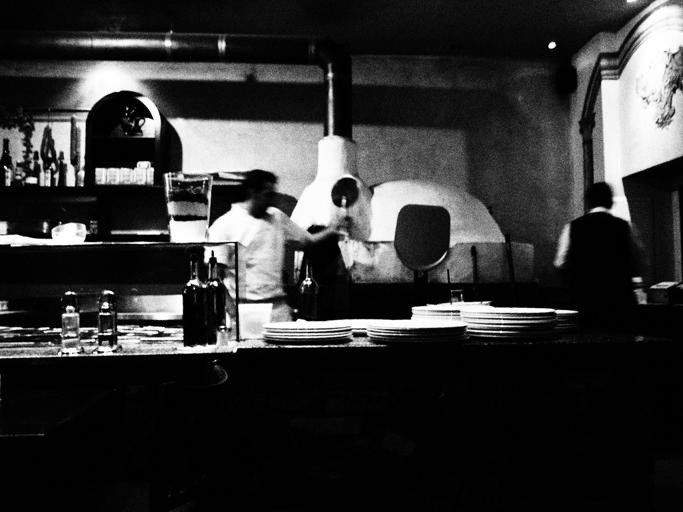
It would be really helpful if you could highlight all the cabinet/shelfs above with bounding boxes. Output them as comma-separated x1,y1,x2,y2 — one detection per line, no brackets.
0,180,251,248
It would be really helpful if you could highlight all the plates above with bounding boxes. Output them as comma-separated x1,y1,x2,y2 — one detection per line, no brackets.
76,324,177,340
261,301,579,345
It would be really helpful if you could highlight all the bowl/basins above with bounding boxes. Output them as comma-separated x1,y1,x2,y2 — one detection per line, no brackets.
50,221,85,244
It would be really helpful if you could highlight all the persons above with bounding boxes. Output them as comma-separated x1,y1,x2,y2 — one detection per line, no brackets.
204,170,348,341
551,182,640,335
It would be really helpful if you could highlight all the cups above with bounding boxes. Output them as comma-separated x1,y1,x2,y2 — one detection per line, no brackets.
0,300,7,310
95,162,153,185
162,171,213,244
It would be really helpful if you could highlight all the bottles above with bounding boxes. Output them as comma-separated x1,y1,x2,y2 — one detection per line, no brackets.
43,162,52,186
180,255,217,345
60,292,80,314
14,160,22,184
298,263,318,316
0,137,13,187
61,305,78,338
203,250,226,328
98,290,117,344
336,196,350,239
449,288,463,301
97,303,114,340
